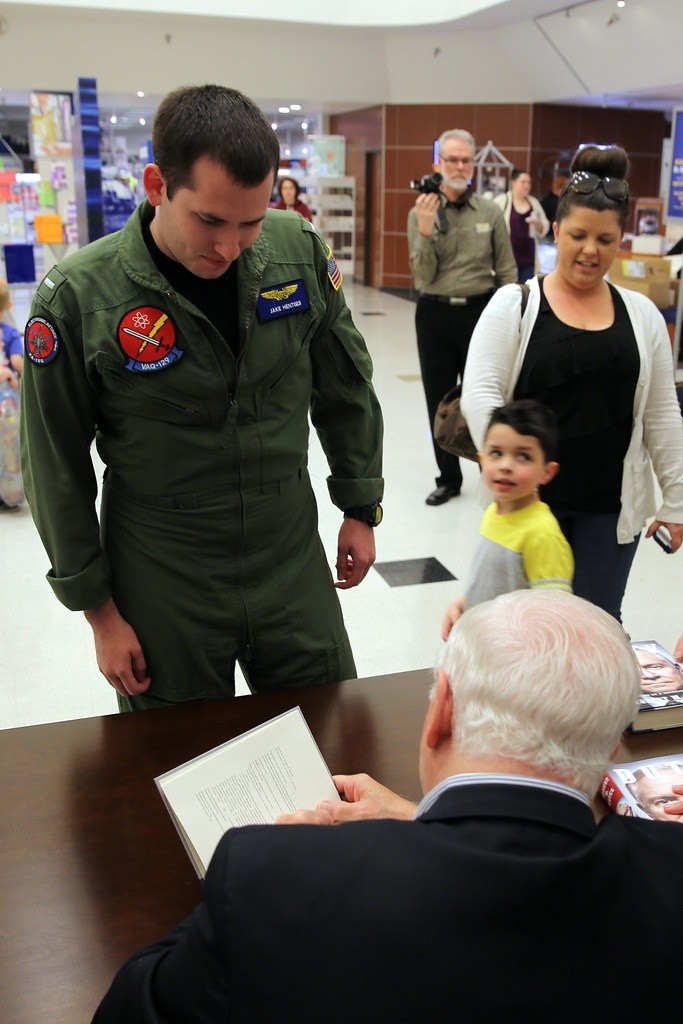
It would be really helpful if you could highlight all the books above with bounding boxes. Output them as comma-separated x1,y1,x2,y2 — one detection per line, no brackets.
598,752,683,821
620,640,683,732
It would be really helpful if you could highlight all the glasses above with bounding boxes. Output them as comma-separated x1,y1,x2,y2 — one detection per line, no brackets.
439,153,475,165
558,171,630,219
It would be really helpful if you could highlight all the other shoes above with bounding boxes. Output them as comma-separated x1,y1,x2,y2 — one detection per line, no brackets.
0,500,20,512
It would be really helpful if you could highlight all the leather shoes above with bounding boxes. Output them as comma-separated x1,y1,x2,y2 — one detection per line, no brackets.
426,487,461,506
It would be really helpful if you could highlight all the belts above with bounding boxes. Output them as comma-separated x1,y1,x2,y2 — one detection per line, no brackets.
419,292,493,306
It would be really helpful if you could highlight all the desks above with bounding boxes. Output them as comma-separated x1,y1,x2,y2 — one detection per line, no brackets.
0,667,683,1024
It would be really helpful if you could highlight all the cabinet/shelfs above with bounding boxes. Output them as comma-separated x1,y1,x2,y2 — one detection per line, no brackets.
298,177,357,280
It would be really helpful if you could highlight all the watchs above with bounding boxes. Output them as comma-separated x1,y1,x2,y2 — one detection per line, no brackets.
345,502,383,528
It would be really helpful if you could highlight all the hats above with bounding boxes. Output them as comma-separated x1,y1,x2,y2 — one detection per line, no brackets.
114,169,130,181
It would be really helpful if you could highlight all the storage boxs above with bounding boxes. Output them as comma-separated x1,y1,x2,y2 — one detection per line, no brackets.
607,251,671,308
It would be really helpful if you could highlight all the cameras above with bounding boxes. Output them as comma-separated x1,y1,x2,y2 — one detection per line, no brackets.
410,172,443,195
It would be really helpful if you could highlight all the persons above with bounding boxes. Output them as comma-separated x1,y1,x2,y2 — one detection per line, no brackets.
21,84,387,716
87,588,683,1024
664,784,683,814
0,279,25,405
618,803,633,816
632,648,683,709
278,129,683,647
626,761,683,823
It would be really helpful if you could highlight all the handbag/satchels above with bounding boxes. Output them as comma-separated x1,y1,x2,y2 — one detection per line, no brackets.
433,384,482,464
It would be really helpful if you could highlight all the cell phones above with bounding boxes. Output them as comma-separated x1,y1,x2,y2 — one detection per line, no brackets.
653,528,672,554
